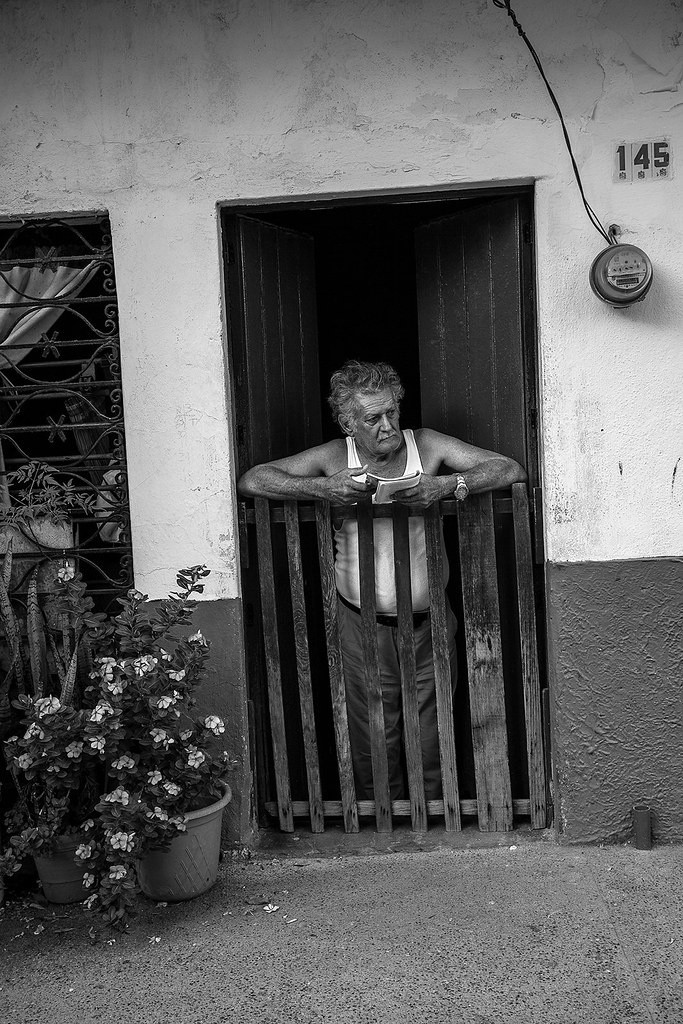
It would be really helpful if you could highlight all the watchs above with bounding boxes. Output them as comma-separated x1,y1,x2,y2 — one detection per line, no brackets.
451,473,470,502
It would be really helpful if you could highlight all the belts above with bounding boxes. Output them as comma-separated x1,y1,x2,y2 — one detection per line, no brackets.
337,593,431,628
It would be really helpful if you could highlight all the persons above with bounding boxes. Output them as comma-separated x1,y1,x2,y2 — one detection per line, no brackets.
239,362,528,807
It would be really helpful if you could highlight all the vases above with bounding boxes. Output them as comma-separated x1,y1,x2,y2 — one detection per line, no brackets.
136,778,233,902
36,833,93,905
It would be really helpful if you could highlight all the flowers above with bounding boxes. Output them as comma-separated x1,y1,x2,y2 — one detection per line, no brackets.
0,564,227,924
